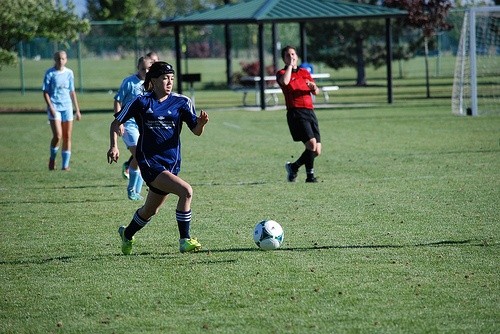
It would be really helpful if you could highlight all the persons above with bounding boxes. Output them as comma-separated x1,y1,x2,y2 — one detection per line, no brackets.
298,60,314,74
276,46,322,183
114,52,159,201
41,50,81,171
106,61,209,254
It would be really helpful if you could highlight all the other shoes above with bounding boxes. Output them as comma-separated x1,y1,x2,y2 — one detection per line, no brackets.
62,167,69,171
122,162,129,179
49,158,56,169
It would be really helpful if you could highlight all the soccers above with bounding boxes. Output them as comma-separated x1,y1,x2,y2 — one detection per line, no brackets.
253,219,284,250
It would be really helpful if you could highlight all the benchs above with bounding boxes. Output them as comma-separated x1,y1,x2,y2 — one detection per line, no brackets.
262,85,339,94
235,87,257,93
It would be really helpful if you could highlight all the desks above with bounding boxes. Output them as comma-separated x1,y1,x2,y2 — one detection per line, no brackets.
237,72,340,106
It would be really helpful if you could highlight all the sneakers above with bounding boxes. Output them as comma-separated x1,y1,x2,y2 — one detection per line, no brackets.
306,178,317,183
127,186,137,200
179,239,201,253
285,161,297,182
136,193,144,200
118,226,134,255
